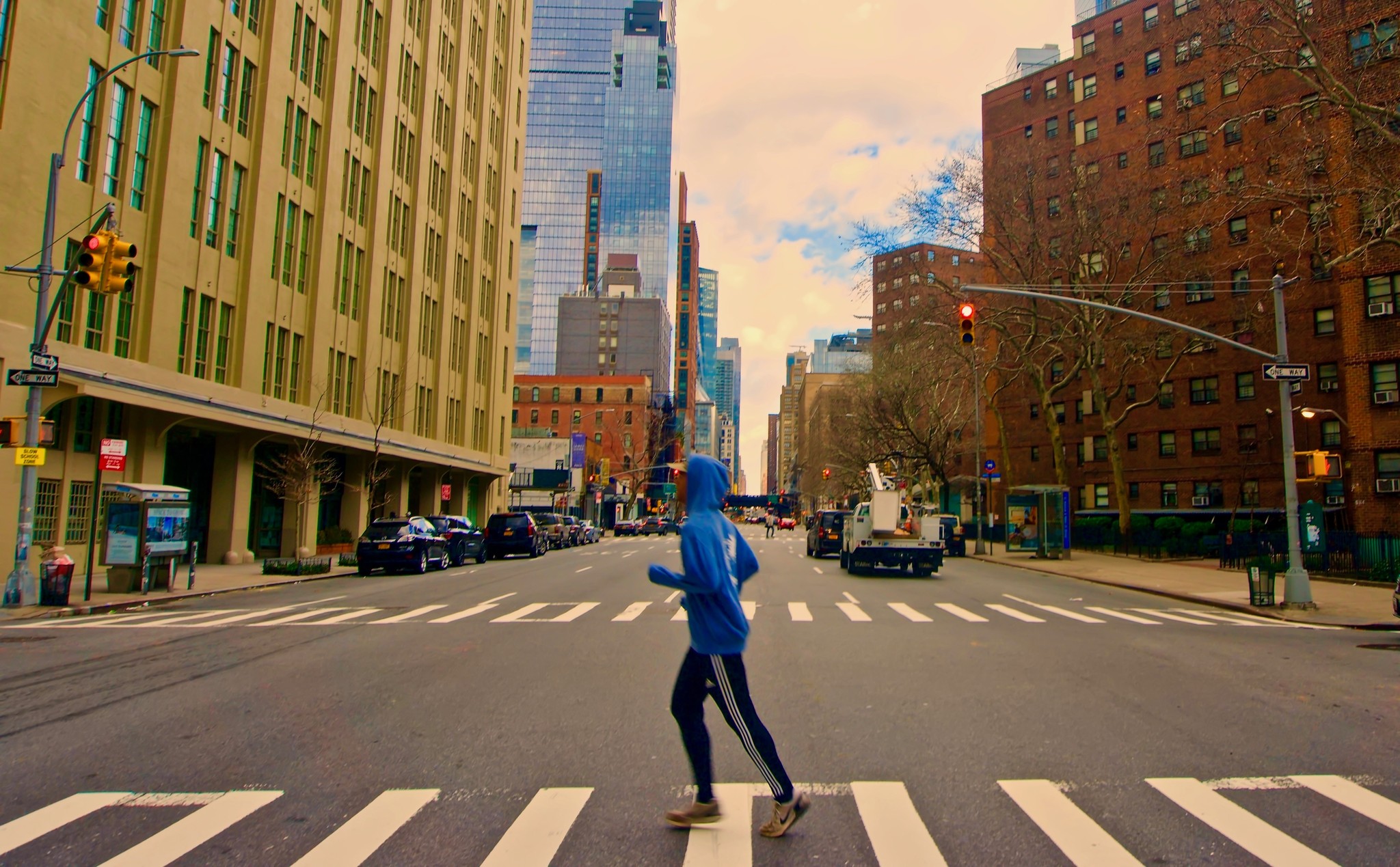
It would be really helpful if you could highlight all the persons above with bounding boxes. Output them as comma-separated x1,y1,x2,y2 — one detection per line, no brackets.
634,517,641,536
405,511,411,517
1014,524,1022,542
648,453,810,837
766,512,774,538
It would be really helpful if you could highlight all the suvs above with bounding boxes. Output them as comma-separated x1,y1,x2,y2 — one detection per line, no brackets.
423,514,490,567
533,512,602,550
483,511,548,560
356,516,453,577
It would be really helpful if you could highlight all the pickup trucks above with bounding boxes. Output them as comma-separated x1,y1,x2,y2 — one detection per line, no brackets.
922,513,966,557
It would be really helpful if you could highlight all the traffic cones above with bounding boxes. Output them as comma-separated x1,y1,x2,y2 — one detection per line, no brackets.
901,510,913,534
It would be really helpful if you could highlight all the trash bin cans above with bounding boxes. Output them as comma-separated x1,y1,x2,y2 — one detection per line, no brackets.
1246,562,1276,606
40,563,75,605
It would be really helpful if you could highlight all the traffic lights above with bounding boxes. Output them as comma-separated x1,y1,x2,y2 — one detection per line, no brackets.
100,234,138,296
74,233,110,292
1324,453,1342,480
958,303,975,346
589,475,595,483
0,420,21,445
673,469,679,480
38,419,56,446
822,469,830,480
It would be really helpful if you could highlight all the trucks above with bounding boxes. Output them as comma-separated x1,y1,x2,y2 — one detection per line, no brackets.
839,462,946,579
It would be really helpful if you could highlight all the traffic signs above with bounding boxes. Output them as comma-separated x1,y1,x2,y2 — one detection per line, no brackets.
30,351,59,372
1261,362,1310,381
5,368,60,388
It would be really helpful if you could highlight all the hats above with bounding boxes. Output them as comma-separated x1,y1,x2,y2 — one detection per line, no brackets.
667,459,687,473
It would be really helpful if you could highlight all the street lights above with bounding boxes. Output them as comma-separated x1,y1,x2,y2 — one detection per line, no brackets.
5,48,202,606
845,412,875,462
923,321,985,556
564,408,615,516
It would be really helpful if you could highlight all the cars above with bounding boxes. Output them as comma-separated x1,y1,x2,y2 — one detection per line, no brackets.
674,515,689,535
642,516,679,536
777,517,796,531
805,509,854,558
613,519,639,536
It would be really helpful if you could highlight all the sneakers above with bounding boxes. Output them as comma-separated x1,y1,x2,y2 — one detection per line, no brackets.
759,791,810,837
666,800,721,827
